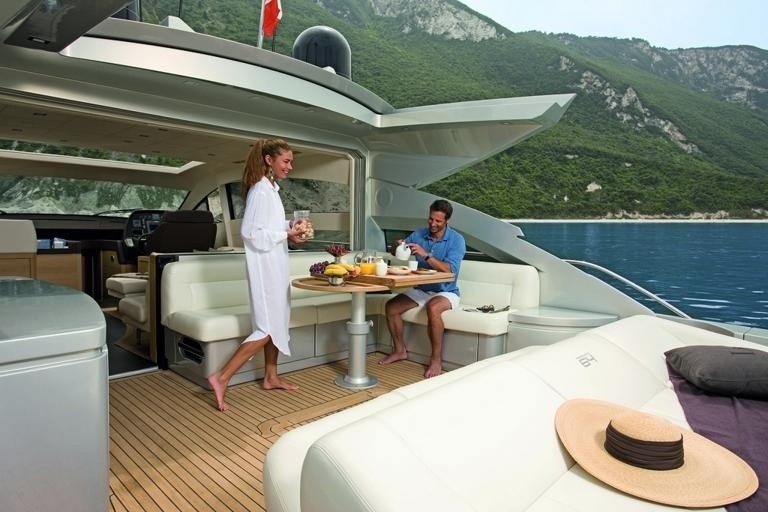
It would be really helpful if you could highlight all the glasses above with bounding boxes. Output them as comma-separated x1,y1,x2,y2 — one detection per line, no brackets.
478,305,494,313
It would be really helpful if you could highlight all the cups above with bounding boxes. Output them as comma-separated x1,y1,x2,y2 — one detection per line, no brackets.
376,263,386,276
409,260,418,271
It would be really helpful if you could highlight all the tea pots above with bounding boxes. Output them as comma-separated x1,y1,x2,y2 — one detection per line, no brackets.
395,241,411,260
354,249,379,273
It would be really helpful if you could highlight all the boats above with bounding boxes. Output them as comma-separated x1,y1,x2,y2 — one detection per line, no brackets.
0,0,767,512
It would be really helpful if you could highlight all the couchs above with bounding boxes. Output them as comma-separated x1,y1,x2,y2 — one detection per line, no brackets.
161,251,538,390
263,315,768,512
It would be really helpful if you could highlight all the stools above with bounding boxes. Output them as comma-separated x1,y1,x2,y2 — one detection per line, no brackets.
505,305,619,353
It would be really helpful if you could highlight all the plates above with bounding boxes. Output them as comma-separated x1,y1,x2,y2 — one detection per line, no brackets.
412,269,437,274
388,270,411,275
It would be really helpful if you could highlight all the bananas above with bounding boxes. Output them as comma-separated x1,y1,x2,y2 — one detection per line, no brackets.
325,263,355,275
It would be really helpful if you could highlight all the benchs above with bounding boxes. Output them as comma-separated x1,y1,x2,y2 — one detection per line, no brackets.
103,271,151,360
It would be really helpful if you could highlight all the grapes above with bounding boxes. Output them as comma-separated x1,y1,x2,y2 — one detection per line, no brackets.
329,246,347,253
310,261,329,274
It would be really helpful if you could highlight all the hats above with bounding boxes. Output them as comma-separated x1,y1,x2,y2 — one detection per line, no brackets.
556,397,759,507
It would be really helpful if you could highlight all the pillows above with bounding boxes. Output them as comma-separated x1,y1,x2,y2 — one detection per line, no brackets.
665,346,768,401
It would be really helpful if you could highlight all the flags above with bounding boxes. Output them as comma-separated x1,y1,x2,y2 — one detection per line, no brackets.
260,0,282,37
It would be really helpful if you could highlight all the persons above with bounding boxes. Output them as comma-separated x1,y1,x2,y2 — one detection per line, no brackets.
378,201,466,380
206,139,310,412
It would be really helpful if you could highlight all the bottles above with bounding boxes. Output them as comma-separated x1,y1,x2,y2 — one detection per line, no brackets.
292,210,315,241
376,257,384,263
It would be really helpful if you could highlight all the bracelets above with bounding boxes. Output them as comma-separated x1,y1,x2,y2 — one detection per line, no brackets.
425,251,432,260
